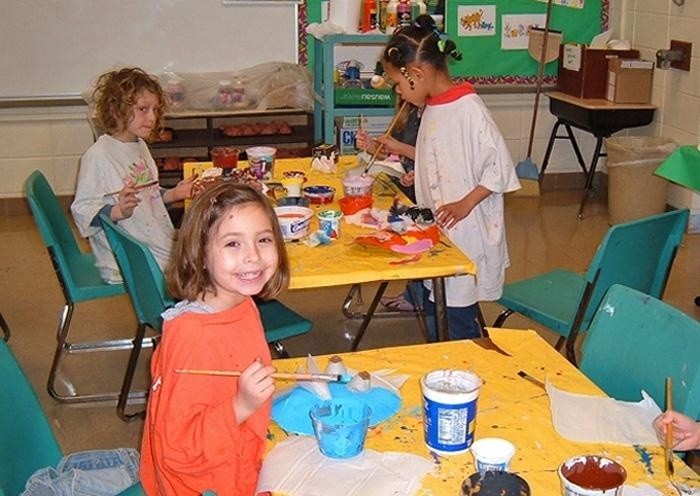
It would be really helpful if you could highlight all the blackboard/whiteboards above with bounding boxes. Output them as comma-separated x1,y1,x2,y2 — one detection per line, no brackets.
0,0,608,107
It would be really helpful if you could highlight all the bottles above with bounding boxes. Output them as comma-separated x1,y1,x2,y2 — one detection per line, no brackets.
370,75,386,88
356,0,427,34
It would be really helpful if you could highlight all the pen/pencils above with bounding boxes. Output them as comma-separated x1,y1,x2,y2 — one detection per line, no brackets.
359,112,364,134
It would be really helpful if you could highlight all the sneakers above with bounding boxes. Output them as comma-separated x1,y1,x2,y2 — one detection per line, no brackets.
378,295,425,311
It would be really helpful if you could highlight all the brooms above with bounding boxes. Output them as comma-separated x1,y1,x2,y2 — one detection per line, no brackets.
511,0,553,199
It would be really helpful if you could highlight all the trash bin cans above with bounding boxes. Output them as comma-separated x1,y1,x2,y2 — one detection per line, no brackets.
602,136,680,226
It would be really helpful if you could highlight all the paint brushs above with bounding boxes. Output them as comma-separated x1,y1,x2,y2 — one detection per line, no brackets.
665,377,674,478
173,369,353,383
365,101,408,173
518,370,546,395
104,180,160,197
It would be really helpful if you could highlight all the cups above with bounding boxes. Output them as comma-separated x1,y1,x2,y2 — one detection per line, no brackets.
461,472,532,496
211,146,241,175
342,176,373,210
316,209,344,241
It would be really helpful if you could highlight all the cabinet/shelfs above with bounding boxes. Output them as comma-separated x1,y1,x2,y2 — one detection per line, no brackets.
140,98,315,196
307,28,451,157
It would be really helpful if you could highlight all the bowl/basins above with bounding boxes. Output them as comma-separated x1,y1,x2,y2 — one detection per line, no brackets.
302,185,336,204
309,397,372,460
270,205,313,240
282,171,306,179
471,437,517,472
276,195,309,207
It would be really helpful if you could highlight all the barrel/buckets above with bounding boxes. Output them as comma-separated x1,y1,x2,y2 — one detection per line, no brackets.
210,147,241,170
341,175,375,198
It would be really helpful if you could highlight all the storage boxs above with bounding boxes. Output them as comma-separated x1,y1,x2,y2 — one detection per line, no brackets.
604,54,657,107
555,40,640,102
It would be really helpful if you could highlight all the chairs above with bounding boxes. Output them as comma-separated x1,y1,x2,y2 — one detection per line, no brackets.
21,164,165,405
1,329,153,496
576,281,700,468
487,205,691,371
96,207,315,428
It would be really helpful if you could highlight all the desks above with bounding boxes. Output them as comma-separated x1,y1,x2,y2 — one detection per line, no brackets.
534,86,657,222
246,327,700,496
172,148,478,356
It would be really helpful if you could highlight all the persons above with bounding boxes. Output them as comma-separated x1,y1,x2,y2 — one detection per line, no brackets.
140,182,289,496
70,67,205,285
371,16,521,343
354,107,425,312
654,411,700,452
